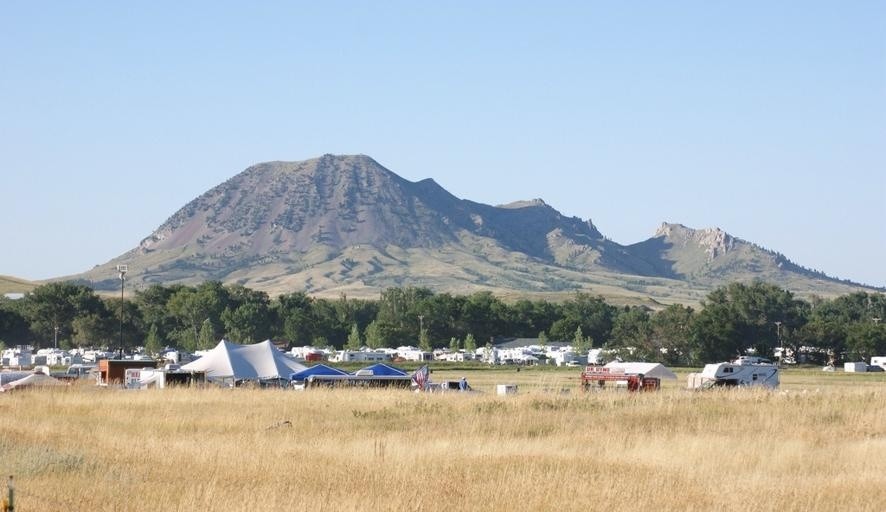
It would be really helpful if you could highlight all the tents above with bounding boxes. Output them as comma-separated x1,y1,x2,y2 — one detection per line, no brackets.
602,360,680,386
174,338,415,389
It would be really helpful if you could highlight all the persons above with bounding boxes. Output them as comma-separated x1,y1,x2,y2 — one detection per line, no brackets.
458,376,469,390
